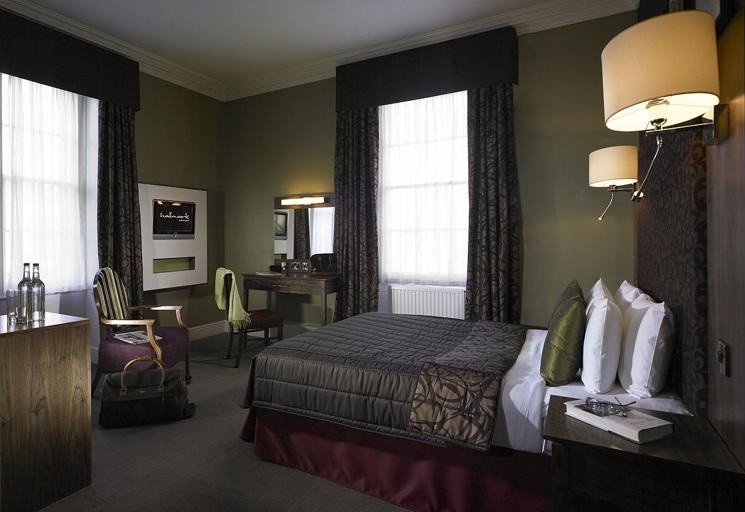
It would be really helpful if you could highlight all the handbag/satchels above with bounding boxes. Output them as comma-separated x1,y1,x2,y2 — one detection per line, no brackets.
98,356,187,430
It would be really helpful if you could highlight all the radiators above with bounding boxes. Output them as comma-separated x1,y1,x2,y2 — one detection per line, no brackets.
390,287,465,320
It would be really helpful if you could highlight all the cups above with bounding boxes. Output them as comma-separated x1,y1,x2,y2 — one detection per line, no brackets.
6,289,18,326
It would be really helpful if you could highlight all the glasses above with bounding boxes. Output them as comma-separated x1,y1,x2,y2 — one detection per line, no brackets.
584,397,636,417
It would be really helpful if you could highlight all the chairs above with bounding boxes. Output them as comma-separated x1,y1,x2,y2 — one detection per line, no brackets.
223,273,285,370
91,265,194,398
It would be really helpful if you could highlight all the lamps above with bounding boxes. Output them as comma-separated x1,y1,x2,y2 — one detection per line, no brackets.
599,8,732,203
586,144,646,220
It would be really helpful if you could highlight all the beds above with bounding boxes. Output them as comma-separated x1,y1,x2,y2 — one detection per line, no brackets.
237,310,694,512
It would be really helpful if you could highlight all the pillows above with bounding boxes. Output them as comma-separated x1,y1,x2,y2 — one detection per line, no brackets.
616,293,676,400
579,276,625,395
613,278,641,314
538,279,587,388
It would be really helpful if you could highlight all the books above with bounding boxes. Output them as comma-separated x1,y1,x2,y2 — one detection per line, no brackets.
564,395,676,445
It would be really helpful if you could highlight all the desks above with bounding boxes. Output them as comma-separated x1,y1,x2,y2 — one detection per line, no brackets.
240,270,338,339
0,307,94,511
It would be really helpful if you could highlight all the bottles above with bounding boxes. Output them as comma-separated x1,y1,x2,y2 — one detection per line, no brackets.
28,263,45,322
17,263,32,325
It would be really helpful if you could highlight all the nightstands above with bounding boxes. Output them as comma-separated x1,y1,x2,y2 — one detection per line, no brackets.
541,394,745,511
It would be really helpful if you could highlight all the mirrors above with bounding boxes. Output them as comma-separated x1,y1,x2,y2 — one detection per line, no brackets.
273,206,335,260
